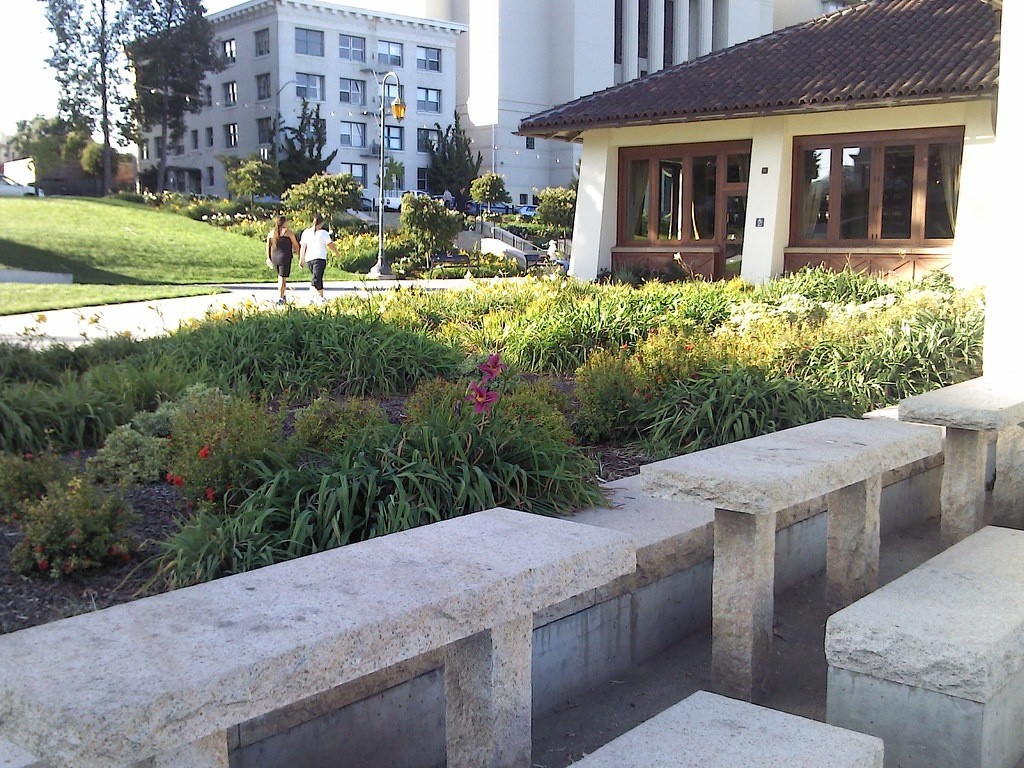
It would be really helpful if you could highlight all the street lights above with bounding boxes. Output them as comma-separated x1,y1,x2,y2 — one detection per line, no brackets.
371,71,407,275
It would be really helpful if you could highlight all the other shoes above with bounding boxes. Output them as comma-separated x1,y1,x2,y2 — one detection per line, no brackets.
320,297,327,303
279,296,288,305
308,300,315,306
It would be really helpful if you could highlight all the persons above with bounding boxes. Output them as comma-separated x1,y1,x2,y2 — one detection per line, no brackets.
299,216,340,303
266,216,299,304
142,186,154,195
548,240,571,277
444,189,452,209
453,186,467,213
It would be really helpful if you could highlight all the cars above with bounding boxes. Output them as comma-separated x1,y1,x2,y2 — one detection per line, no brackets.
513,205,538,217
465,200,517,215
0,176,44,198
433,195,455,209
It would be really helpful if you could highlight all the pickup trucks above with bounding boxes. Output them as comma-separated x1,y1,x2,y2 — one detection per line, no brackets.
373,190,432,212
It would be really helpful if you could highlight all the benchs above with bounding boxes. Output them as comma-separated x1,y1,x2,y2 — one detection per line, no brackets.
524,254,550,275
0,506,638,768
824,523,1024,767
897,376,1024,544
638,416,943,706
428,247,469,267
560,687,887,768
